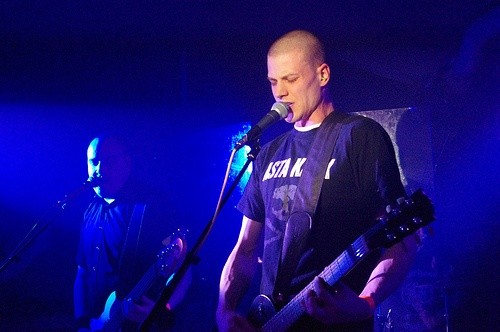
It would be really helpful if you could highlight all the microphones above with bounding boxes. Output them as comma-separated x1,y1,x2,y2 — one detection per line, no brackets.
234,102,290,151
61,177,96,202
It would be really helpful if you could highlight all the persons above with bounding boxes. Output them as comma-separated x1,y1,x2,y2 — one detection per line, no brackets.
74,132,192,332
215,30,418,332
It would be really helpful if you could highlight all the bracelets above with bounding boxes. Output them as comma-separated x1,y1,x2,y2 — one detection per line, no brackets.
356,294,376,322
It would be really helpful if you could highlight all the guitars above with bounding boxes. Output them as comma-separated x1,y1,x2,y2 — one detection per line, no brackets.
73,223,192,330
244,186,437,329
